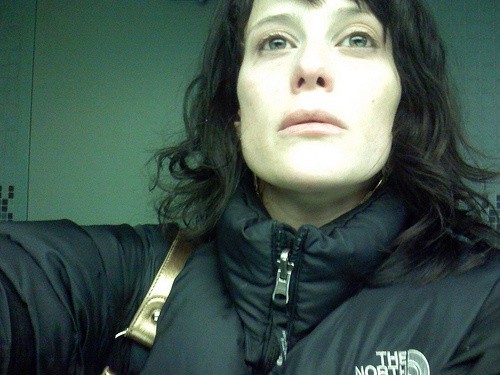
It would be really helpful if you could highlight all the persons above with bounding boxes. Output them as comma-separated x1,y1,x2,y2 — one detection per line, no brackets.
0,1,499,374
